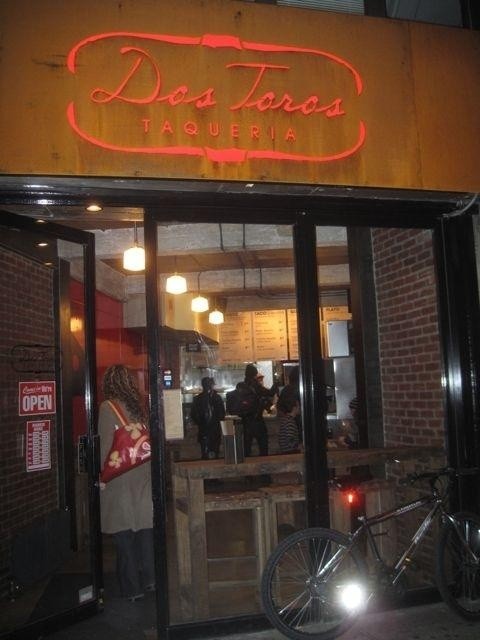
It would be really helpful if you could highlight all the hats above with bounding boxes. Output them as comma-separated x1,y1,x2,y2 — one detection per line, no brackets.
246,364,257,376
201,377,216,385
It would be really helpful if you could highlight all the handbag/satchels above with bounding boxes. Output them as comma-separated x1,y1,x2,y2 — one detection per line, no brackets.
101,422,151,482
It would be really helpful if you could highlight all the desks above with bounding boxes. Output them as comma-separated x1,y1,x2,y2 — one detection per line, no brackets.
172,444,447,622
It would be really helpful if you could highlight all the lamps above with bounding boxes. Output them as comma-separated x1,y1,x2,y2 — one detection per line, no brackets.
121,222,225,326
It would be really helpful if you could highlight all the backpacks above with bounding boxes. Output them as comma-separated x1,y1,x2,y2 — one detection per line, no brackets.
190,391,218,424
226,382,261,414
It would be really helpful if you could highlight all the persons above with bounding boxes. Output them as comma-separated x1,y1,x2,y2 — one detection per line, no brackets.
340,397,358,448
277,397,304,456
278,364,300,439
98,361,161,605
238,365,279,455
191,377,225,460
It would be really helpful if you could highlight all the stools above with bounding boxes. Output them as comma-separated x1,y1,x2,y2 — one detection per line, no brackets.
262,484,347,609
173,488,266,622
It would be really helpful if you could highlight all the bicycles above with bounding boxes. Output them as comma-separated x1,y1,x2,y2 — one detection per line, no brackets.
261,462,479,639
